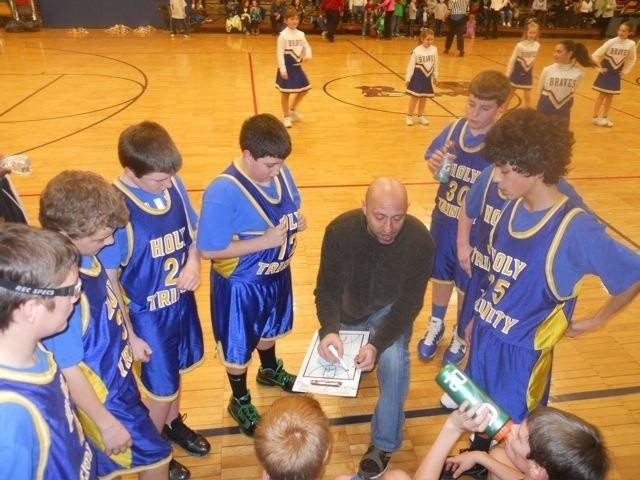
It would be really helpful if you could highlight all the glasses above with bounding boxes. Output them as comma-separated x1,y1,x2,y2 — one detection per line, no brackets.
0,277,83,298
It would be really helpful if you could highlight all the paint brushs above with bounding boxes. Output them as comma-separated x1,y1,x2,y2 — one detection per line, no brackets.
328,345,349,373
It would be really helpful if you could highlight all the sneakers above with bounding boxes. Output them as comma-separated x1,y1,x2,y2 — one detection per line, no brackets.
440,448,489,480
416,315,446,363
507,21,512,27
406,116,413,126
163,412,211,457
441,323,468,367
168,459,191,480
444,48,449,54
459,50,464,57
288,109,304,122
418,116,430,126
357,442,393,479
283,116,293,128
502,21,507,27
227,388,262,437
256,358,305,394
602,117,614,127
591,117,607,126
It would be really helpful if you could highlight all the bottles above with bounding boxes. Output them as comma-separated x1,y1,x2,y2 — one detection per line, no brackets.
434,139,457,183
0,155,32,176
434,363,515,444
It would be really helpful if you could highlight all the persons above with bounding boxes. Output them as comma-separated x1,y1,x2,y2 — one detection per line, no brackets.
537,40,599,121
0,226,96,479
315,177,436,478
158,0,207,36
592,22,637,128
440,106,640,474
270,0,304,34
313,0,448,43
376,400,610,480
0,153,31,224
274,6,312,127
500,19,540,112
417,72,510,366
466,0,640,38
197,114,307,438
444,1,470,58
253,391,359,480
404,29,439,126
223,0,261,35
39,169,173,480
98,122,210,480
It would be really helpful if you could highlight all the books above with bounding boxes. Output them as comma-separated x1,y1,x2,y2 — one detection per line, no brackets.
292,329,370,397
